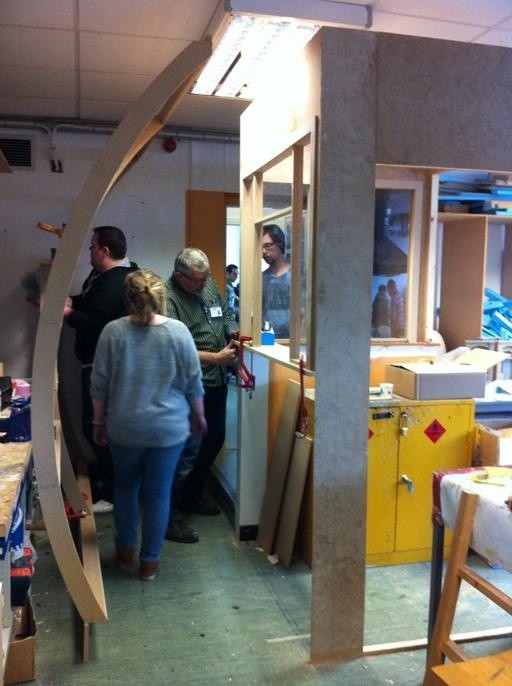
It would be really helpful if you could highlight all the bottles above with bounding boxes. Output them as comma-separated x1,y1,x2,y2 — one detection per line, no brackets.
264,320,274,332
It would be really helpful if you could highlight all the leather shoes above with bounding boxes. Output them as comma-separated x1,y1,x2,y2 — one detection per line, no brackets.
180,494,222,516
164,518,199,544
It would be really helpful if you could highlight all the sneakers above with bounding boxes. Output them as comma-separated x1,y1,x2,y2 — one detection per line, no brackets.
92,498,115,514
115,547,136,573
142,557,161,581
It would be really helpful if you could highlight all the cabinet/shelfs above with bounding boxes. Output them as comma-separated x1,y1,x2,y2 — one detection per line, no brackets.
432,166,512,352
300,383,479,572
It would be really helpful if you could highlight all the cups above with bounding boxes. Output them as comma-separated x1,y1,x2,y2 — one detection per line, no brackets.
380,382,393,399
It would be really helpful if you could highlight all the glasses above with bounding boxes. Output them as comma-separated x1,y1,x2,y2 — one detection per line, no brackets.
180,273,207,287
262,241,277,248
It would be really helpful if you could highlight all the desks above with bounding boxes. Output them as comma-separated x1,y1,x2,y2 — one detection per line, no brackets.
0,402,37,686
428,465,512,668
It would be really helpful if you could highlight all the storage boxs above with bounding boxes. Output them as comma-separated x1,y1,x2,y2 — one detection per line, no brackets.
471,416,511,467
384,343,511,401
3,592,38,686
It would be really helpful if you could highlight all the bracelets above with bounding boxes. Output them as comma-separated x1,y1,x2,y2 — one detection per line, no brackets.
91,421,105,426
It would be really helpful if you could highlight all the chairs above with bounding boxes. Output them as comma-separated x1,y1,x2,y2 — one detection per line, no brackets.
419,484,512,685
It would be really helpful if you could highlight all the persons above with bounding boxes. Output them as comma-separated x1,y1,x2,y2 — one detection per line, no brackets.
387,278,405,337
165,247,240,545
372,283,390,333
226,265,240,322
91,269,208,583
63,226,135,513
263,224,291,338
